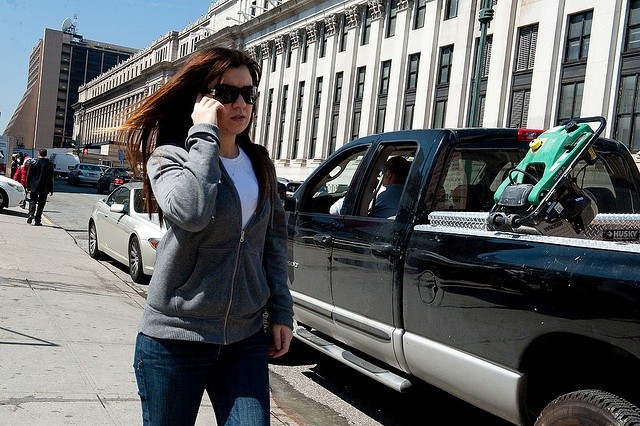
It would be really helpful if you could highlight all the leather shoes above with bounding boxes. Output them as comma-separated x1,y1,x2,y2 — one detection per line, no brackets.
35,221,42,225
27,215,34,223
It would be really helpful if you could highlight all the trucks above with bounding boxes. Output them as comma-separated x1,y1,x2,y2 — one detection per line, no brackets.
50,153,80,180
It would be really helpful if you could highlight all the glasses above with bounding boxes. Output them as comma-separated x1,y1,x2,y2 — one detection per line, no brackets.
199,83,258,104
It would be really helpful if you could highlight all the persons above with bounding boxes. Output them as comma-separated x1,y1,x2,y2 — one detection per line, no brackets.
24,148,54,226
13,155,36,187
115,45,295,425
366,155,411,219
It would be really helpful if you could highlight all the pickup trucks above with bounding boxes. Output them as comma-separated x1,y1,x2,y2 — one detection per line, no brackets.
264,127,640,426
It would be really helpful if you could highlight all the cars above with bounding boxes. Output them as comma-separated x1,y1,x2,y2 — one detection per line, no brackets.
98,165,111,172
68,163,104,186
98,167,134,194
88,181,169,284
0,174,26,211
